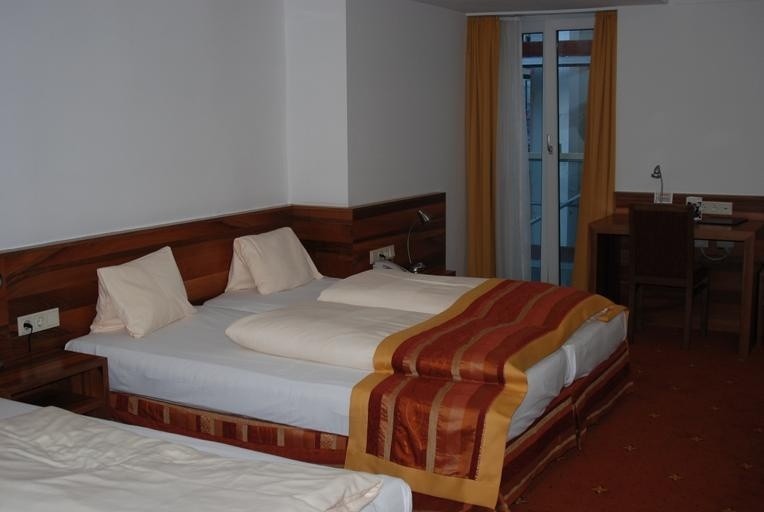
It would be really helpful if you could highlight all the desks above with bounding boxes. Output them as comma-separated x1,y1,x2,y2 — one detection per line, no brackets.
585,213,764,360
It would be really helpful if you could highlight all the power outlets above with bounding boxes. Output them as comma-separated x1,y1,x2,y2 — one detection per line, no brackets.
369,244,396,265
16,308,62,337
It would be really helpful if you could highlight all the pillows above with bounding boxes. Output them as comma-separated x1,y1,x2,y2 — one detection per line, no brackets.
91,226,323,337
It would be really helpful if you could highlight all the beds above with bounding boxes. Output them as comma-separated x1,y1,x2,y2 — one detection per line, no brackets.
67,269,629,510
2,396,413,512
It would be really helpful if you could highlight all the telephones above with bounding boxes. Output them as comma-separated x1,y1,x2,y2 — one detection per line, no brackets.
372,259,412,274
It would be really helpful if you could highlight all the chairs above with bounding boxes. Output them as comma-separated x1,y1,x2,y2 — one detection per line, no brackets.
627,202,714,352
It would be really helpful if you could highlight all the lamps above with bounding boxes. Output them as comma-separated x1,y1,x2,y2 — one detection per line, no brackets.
406,208,434,272
651,163,665,205
